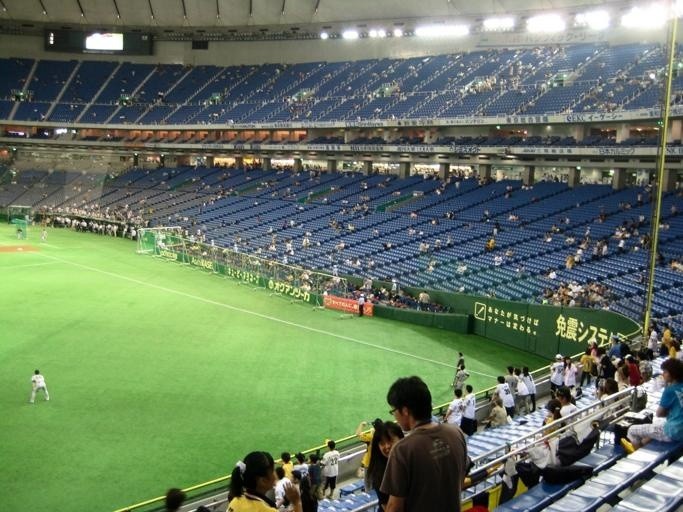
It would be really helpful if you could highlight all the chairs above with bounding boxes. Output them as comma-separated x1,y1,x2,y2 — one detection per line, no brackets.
135,62,316,306
317,42,682,336
298,335,683,512
1,56,136,241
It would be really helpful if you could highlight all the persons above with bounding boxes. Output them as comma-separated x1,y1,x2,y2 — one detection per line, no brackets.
30,370,50,403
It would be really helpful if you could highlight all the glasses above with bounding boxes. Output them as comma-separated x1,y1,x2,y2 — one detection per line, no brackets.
389,407,396,415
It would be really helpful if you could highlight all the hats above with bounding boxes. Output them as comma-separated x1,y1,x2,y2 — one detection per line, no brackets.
554,354,562,360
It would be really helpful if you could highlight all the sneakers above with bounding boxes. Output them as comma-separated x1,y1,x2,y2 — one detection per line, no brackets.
620,437,634,453
